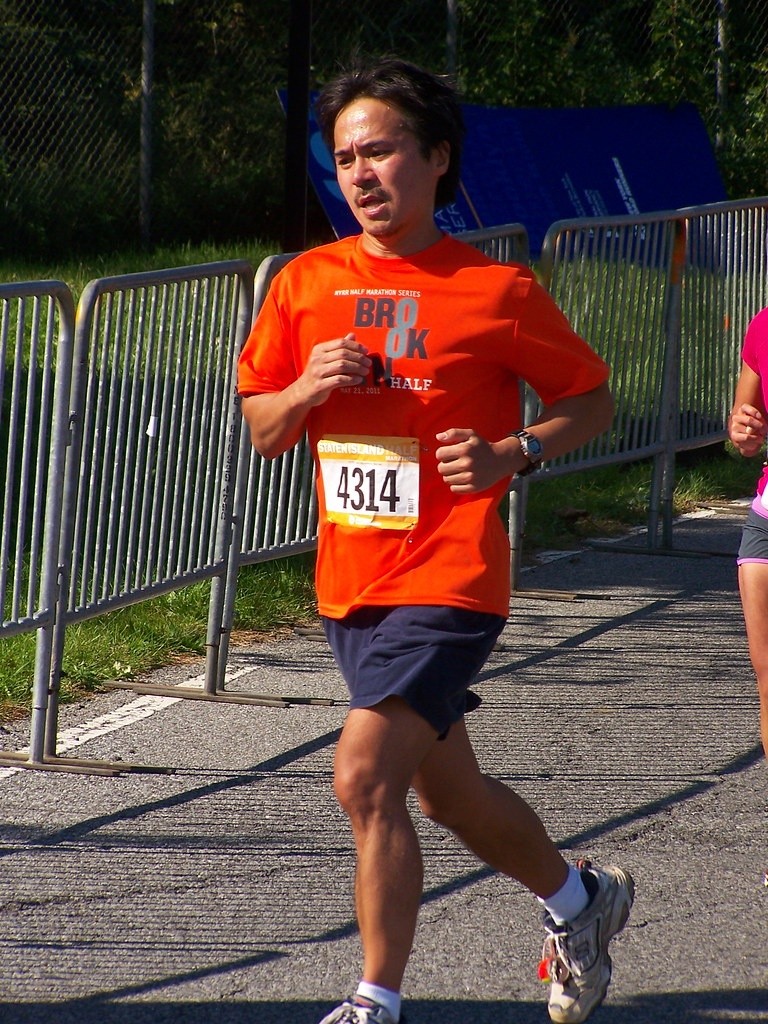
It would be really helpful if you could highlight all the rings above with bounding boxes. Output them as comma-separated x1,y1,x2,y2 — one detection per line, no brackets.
745,426,753,434
747,417,754,427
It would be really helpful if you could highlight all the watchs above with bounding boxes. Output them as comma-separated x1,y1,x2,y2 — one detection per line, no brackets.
508,428,545,479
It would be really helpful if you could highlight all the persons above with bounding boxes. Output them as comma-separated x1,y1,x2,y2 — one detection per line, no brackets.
229,64,635,1024
724,304,768,762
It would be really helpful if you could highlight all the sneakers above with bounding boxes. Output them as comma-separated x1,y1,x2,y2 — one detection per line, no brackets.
318,991,399,1024
543,856,636,1023
